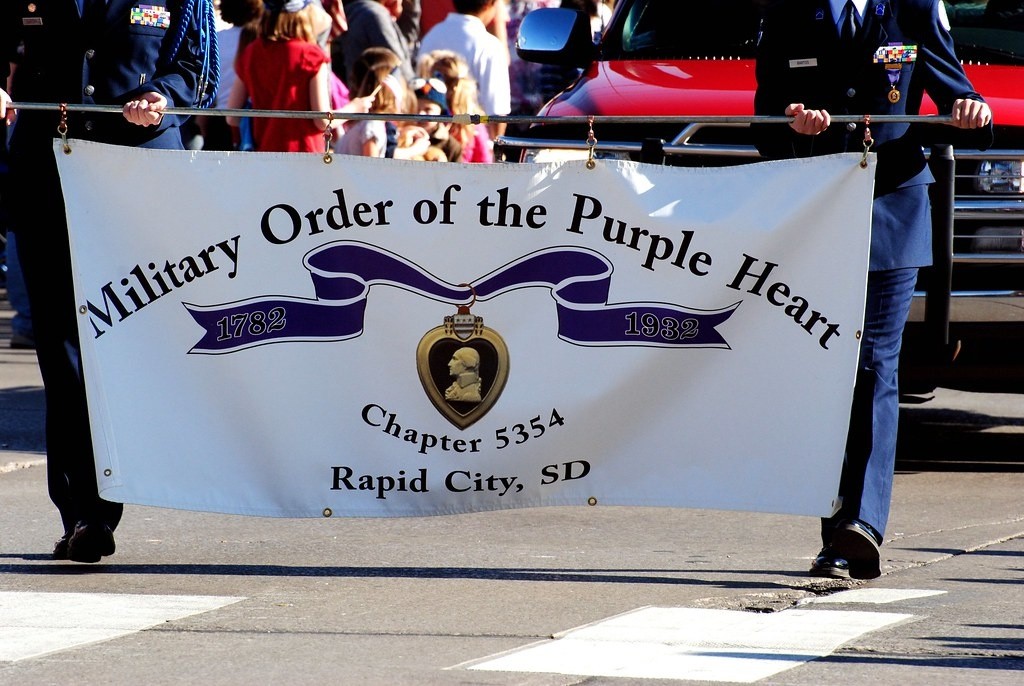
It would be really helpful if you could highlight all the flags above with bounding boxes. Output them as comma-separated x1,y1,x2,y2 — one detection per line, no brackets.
51,136,878,519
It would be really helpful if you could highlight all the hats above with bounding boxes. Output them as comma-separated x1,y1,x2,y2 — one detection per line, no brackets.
263,0,310,12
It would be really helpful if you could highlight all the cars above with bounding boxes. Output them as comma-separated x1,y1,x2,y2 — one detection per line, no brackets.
489,0,1024,402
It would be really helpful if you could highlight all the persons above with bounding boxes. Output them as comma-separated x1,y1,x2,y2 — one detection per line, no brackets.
225,0,376,155
208,0,264,149
325,0,508,161
1,0,220,562
444,347,482,402
749,0,992,581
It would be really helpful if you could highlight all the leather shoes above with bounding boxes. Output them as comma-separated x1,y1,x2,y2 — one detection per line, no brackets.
810,517,884,580
51,514,116,563
